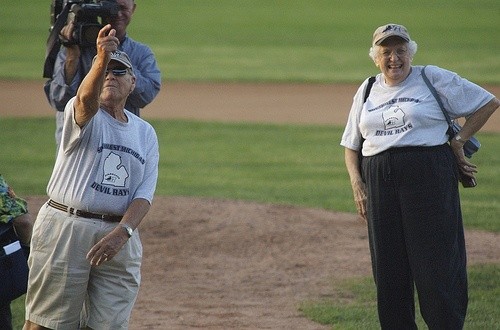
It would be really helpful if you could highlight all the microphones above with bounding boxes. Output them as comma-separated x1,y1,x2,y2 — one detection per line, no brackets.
71,3,86,18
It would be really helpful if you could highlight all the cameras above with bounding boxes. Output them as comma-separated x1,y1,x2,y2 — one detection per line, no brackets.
459,166,477,188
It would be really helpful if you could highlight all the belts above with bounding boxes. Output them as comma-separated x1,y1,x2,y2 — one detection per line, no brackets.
49,199,124,222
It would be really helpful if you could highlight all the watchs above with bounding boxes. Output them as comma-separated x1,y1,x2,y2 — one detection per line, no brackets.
455,133,469,143
119,223,133,238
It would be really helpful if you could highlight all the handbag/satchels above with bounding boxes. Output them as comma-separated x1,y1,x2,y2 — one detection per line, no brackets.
448,122,481,158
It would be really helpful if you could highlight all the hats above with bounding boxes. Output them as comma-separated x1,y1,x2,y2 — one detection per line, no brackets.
91,51,133,70
372,24,410,45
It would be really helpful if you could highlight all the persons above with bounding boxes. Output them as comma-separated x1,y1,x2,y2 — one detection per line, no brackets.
22,24,159,330
44,0,161,157
339,24,500,330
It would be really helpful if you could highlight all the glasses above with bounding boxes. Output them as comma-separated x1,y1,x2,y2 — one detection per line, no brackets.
380,46,409,59
105,67,128,76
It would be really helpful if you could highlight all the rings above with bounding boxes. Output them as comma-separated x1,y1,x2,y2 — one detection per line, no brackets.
103,253,109,257
